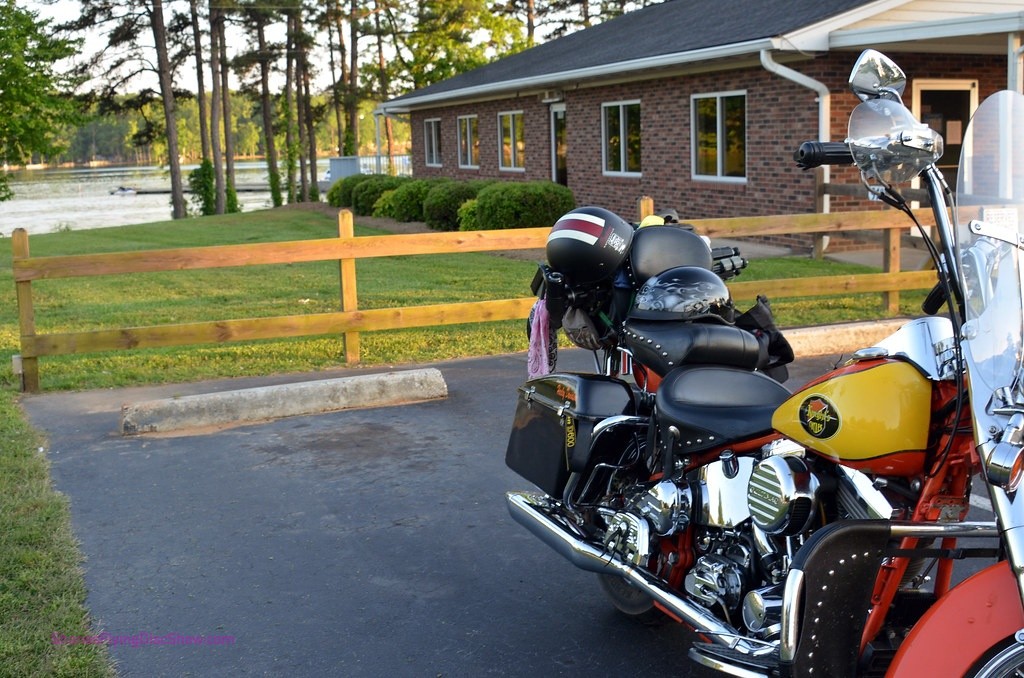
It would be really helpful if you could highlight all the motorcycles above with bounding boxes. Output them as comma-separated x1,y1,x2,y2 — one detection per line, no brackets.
502,49,1024,678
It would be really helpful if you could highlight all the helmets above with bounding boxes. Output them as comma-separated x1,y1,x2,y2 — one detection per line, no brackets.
546,205,635,286
